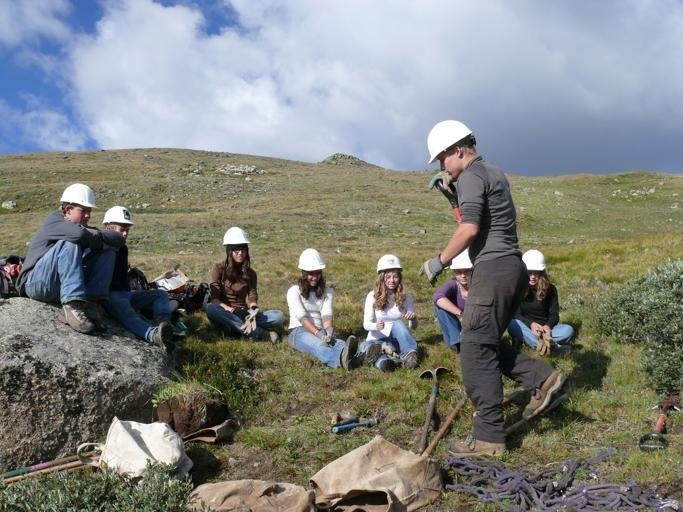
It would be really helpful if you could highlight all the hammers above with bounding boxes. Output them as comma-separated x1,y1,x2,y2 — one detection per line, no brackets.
332,417,380,431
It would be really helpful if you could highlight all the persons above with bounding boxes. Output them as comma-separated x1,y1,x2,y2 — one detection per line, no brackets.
285,248,382,371
11,181,115,336
506,249,575,356
359,254,423,372
433,248,475,355
86,206,181,355
204,226,285,345
416,117,566,459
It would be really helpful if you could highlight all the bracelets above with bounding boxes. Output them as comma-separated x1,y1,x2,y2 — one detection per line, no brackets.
456,311,464,320
314,330,319,336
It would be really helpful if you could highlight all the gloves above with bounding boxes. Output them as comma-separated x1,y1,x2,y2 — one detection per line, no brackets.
241,308,260,335
316,326,336,348
535,327,555,357
428,170,456,190
418,254,452,287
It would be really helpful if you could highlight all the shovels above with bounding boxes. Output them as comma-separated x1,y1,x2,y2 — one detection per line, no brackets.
639,402,680,450
420,366,453,454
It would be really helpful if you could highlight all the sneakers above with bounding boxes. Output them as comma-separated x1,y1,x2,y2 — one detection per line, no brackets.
153,317,186,353
58,303,109,334
341,335,358,371
353,343,418,371
447,435,508,458
521,368,567,420
262,329,280,344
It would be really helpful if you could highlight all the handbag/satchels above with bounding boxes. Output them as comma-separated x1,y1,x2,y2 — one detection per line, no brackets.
188,479,308,512
309,436,445,512
100,416,186,483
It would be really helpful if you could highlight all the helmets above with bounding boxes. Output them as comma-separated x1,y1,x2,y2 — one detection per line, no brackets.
223,227,251,245
522,250,546,271
103,206,134,225
377,254,403,272
427,120,473,164
298,249,326,271
450,248,474,269
60,184,98,209
